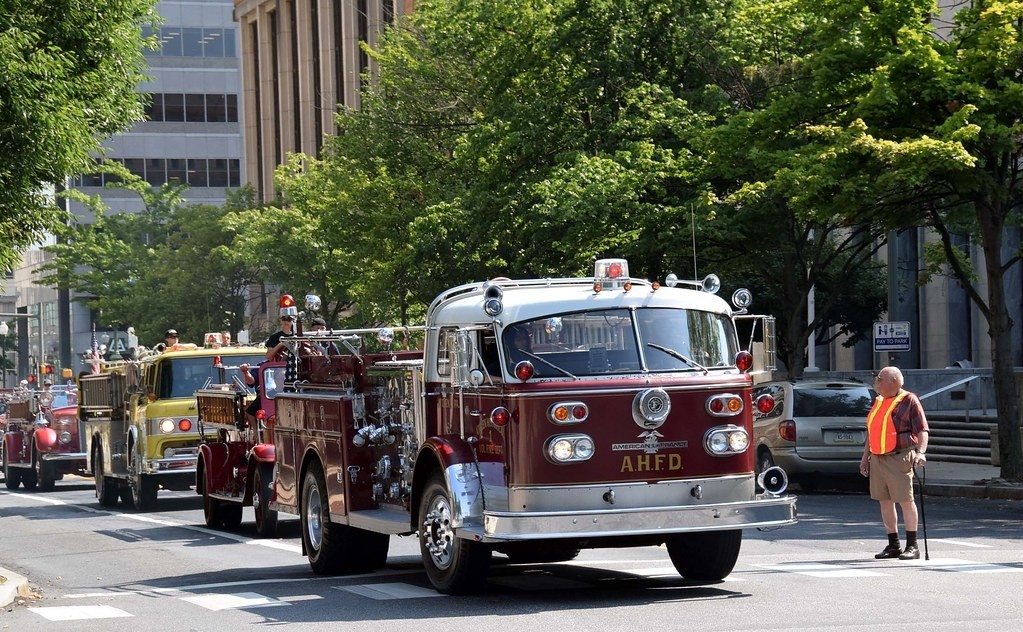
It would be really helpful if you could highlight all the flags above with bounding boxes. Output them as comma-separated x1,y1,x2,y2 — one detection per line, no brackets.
90,330,101,374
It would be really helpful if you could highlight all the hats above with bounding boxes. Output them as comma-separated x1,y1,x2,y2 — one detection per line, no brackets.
311,317,326,326
43,379,53,385
164,328,180,336
279,306,298,317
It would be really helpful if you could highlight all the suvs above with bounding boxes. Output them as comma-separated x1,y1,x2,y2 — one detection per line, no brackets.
749,378,879,488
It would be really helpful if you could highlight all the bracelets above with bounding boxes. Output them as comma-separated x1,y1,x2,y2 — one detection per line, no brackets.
917,450,925,454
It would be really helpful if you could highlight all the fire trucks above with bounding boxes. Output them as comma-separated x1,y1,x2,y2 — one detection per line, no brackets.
79,342,268,511
0,384,82,492
194,257,799,594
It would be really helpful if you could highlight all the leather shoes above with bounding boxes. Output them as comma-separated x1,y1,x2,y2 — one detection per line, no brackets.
899,546,920,560
875,545,904,560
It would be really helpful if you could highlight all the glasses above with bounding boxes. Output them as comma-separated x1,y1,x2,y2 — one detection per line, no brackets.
281,317,292,322
877,375,892,381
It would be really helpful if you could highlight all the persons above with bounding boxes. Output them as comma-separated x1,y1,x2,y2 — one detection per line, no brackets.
62,391,76,406
265,315,295,361
307,317,340,356
221,331,231,347
860,366,929,559
164,329,197,350
43,379,52,391
239,340,287,443
508,325,532,364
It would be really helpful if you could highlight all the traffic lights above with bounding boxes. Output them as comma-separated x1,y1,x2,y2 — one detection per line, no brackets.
46,365,53,375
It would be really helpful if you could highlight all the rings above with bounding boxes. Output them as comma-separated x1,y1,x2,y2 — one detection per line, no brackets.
915,461,918,463
